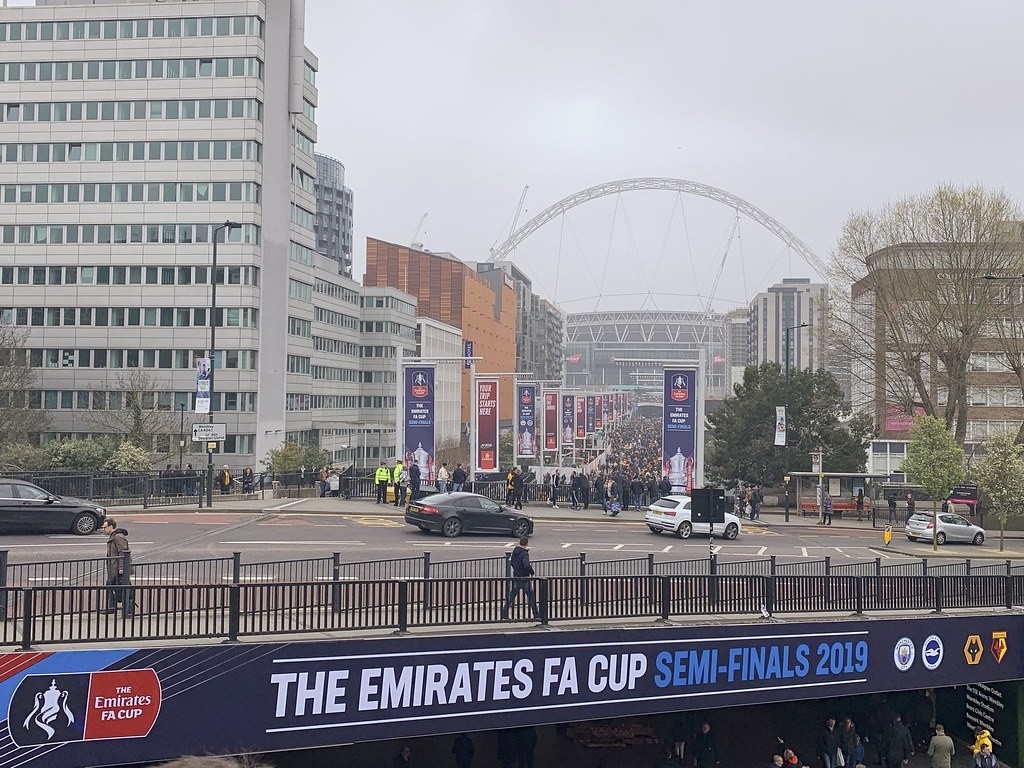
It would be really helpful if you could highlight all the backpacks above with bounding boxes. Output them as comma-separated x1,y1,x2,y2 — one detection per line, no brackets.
942,501,949,512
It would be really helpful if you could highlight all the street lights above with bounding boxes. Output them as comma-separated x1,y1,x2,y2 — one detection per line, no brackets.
785,322,814,523
206,220,242,505
340,444,355,473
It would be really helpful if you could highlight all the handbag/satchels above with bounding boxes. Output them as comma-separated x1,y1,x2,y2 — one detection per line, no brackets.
745,504,752,514
116,580,136,603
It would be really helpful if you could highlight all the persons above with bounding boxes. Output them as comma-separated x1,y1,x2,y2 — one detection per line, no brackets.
942,500,955,513
503,538,539,619
438,463,466,494
196,361,210,382
101,519,132,616
823,494,833,525
543,469,672,517
218,465,231,494
506,467,524,509
244,468,254,493
300,464,339,498
888,494,898,523
392,727,537,768
738,489,763,520
855,489,864,521
375,460,421,507
596,413,662,481
655,689,1000,768
906,494,915,521
163,463,197,497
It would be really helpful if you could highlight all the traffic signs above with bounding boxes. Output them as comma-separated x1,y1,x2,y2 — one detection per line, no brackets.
191,423,227,442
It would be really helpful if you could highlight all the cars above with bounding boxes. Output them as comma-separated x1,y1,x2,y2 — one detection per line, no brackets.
644,496,743,539
405,491,534,537
0,478,107,535
903,511,987,547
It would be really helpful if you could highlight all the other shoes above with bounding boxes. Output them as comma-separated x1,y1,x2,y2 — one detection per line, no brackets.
394,504,398,506
376,501,380,504
399,504,405,507
99,609,115,615
827,523,830,525
553,504,559,509
603,511,606,514
383,501,388,504
547,498,550,505
577,505,580,511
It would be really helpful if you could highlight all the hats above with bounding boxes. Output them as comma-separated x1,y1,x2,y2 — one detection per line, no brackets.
381,462,386,465
397,460,402,464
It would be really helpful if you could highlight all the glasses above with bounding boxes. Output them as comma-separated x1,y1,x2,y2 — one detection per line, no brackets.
101,525,110,529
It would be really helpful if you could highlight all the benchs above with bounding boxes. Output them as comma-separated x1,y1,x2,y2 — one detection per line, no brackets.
802,503,870,520
